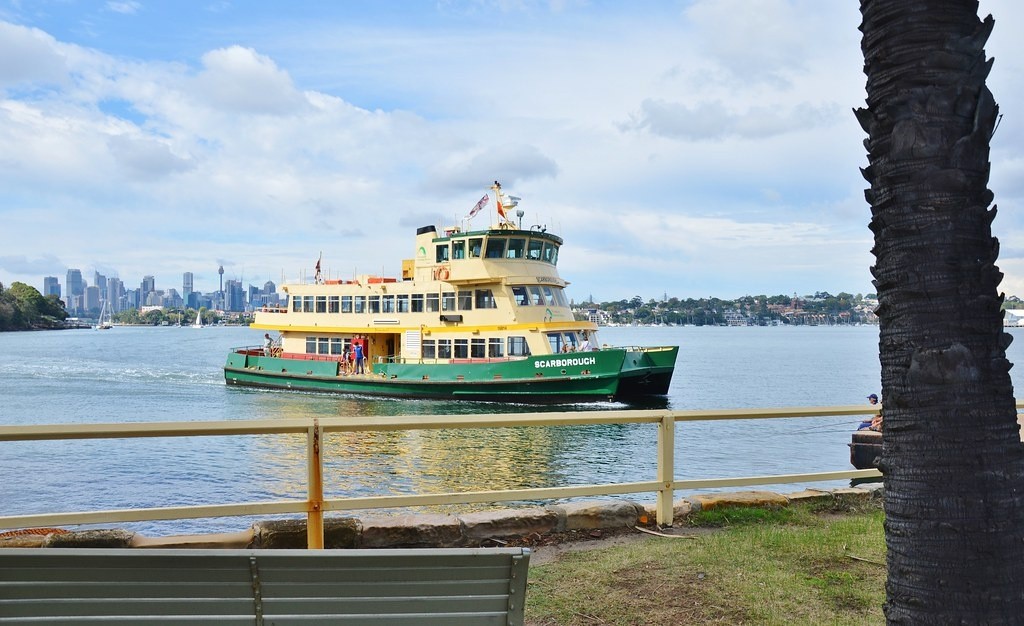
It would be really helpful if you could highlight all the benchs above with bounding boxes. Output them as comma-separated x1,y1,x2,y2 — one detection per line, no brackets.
0,548,533,626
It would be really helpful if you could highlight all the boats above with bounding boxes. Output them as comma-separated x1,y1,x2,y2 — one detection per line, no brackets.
223,179,682,406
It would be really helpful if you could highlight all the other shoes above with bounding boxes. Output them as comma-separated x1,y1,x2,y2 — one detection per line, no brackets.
350,371,354,375
361,371,364,374
356,371,359,374
344,372,347,376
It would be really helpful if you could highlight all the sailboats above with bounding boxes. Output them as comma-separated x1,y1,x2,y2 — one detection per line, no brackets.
90,299,114,330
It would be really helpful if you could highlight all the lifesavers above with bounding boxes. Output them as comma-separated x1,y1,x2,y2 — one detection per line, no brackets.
436,265,449,281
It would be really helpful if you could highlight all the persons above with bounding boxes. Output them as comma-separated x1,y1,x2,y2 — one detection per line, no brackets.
273,303,279,312
264,334,274,357
354,342,364,374
562,344,577,353
262,304,267,312
473,245,481,257
580,337,591,352
342,349,348,375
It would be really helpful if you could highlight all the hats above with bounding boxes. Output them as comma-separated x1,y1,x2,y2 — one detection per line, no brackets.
867,394,878,399
